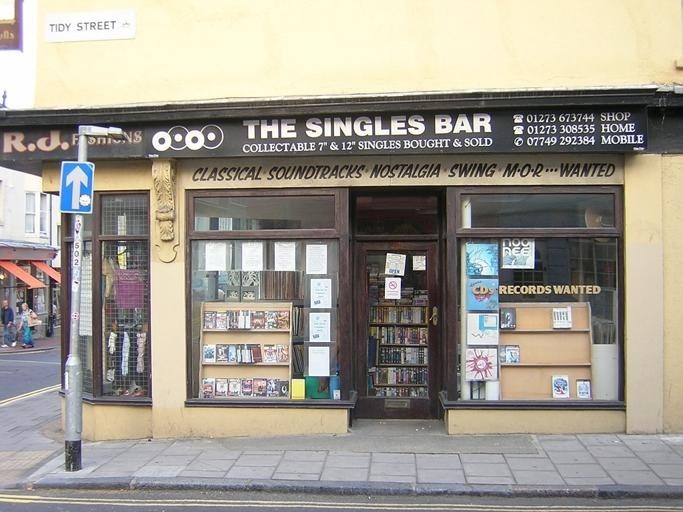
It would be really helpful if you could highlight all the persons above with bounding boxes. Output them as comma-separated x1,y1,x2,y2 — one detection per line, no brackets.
17,302,38,349
0,298,18,348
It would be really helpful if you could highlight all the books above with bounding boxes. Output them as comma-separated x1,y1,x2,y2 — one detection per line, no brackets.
500,307,516,330
552,307,572,330
198,270,307,401
499,344,520,363
505,347,520,364
576,378,592,401
366,270,429,398
551,374,569,399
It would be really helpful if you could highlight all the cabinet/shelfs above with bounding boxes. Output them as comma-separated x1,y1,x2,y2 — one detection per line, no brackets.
191,234,616,400
